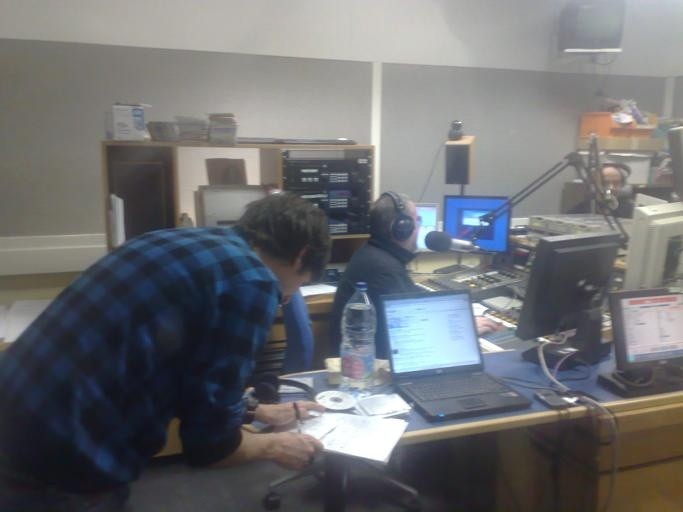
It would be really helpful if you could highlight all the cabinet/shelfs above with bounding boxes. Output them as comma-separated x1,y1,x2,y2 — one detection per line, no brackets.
100,137,375,368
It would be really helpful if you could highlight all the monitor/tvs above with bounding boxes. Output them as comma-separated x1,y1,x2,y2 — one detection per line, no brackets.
199,186,269,229
414,203,439,253
560,0,625,49
623,201,683,290
598,286,683,398
515,231,621,370
443,196,511,254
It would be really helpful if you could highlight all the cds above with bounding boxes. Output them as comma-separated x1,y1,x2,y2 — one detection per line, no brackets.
315,390,356,410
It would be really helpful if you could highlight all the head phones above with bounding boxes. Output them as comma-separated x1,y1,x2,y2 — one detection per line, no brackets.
381,190,414,240
602,162,631,188
254,374,317,406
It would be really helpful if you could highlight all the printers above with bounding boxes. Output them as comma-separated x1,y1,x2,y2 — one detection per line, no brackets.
195,159,246,229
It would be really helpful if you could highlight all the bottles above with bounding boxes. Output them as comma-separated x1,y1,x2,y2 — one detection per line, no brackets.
339,281,377,398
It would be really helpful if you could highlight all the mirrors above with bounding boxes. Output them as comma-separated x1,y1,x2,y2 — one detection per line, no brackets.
177,146,261,228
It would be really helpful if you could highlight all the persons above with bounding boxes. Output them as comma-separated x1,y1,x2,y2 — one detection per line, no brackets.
570,165,634,218
322,192,501,358
1,191,334,511
257,281,315,377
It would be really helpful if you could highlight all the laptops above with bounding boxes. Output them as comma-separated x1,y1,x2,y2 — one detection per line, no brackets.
379,289,531,422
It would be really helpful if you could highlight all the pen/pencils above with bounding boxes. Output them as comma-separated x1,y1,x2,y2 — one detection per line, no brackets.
296,419,301,436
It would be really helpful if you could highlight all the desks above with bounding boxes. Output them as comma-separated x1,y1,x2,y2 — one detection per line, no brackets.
240,350,683,512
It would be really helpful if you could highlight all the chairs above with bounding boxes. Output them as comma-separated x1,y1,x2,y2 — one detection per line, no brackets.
262,288,428,512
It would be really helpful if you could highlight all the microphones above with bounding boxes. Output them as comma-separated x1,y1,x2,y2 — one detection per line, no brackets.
605,189,619,210
425,232,480,253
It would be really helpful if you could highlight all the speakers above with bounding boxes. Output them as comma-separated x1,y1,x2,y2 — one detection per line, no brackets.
445,135,475,184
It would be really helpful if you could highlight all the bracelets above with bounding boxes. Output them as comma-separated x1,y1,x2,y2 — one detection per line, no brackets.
245,395,259,421
